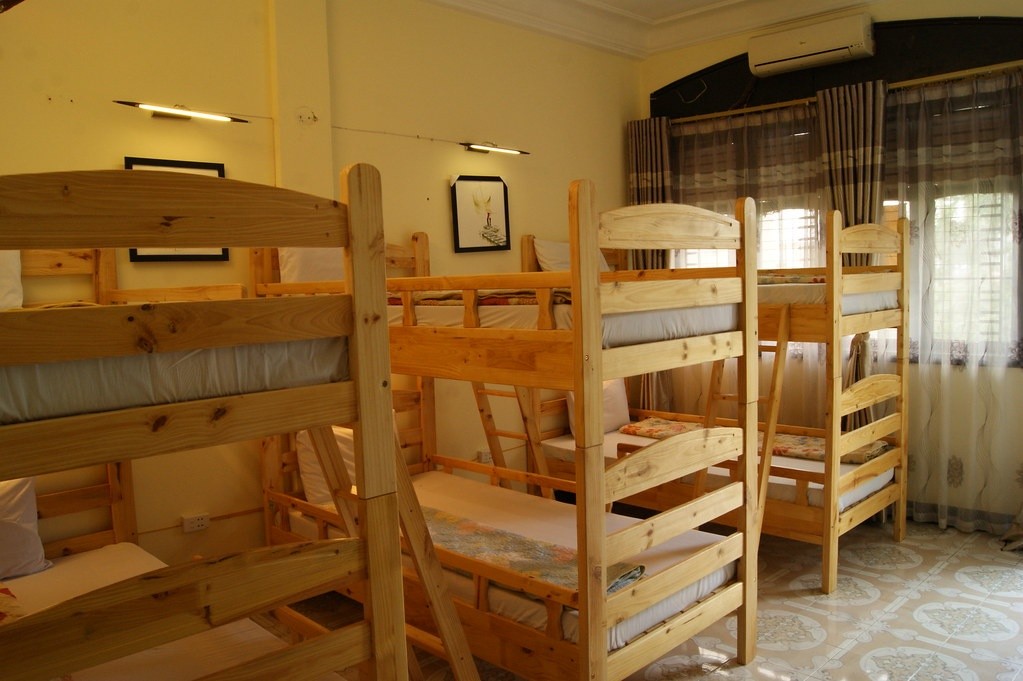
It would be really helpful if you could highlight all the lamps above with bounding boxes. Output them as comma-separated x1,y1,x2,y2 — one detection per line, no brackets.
113,100,250,124
459,141,530,157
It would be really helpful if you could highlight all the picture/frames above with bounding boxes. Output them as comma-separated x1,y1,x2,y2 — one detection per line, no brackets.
450,174,511,253
125,156,230,262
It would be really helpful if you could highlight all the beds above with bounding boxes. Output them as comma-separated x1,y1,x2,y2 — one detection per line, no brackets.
0,160,918,681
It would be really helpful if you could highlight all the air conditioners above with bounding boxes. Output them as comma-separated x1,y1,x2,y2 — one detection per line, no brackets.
747,13,874,79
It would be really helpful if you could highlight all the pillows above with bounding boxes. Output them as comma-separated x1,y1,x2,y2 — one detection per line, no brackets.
277,247,343,283
0,250,23,309
531,236,611,275
0,476,54,582
566,377,631,440
295,425,356,502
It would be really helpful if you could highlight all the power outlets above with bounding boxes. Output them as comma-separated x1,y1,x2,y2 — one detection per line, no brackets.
180,513,211,535
478,450,490,464
